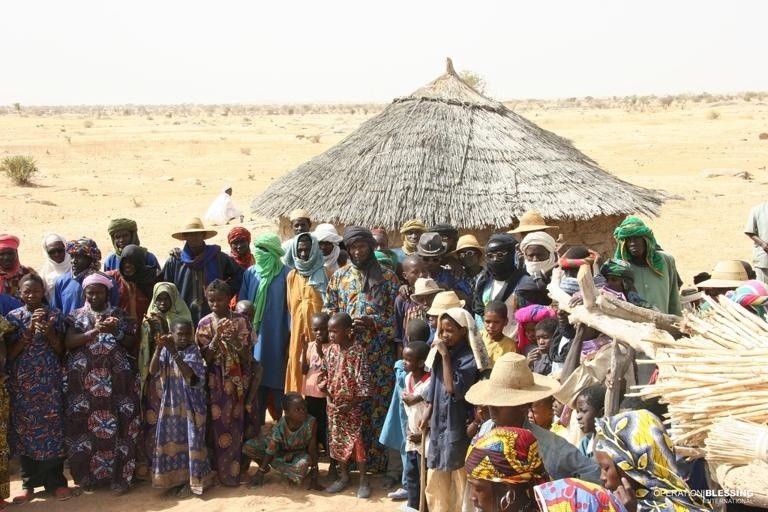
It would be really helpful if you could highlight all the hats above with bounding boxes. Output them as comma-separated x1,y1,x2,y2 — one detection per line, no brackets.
427,291,465,316
410,278,445,304
697,260,749,288
172,218,217,240
465,352,561,407
290,210,311,221
416,232,448,256
506,211,559,234
448,234,486,259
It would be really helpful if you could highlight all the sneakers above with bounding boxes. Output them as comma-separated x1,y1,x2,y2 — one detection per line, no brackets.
14,491,33,503
326,479,350,493
358,486,372,498
388,487,410,499
54,488,72,501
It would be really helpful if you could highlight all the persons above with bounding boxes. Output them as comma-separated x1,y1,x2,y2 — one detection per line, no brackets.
745,198,768,287
0,209,767,512
204,184,241,224
